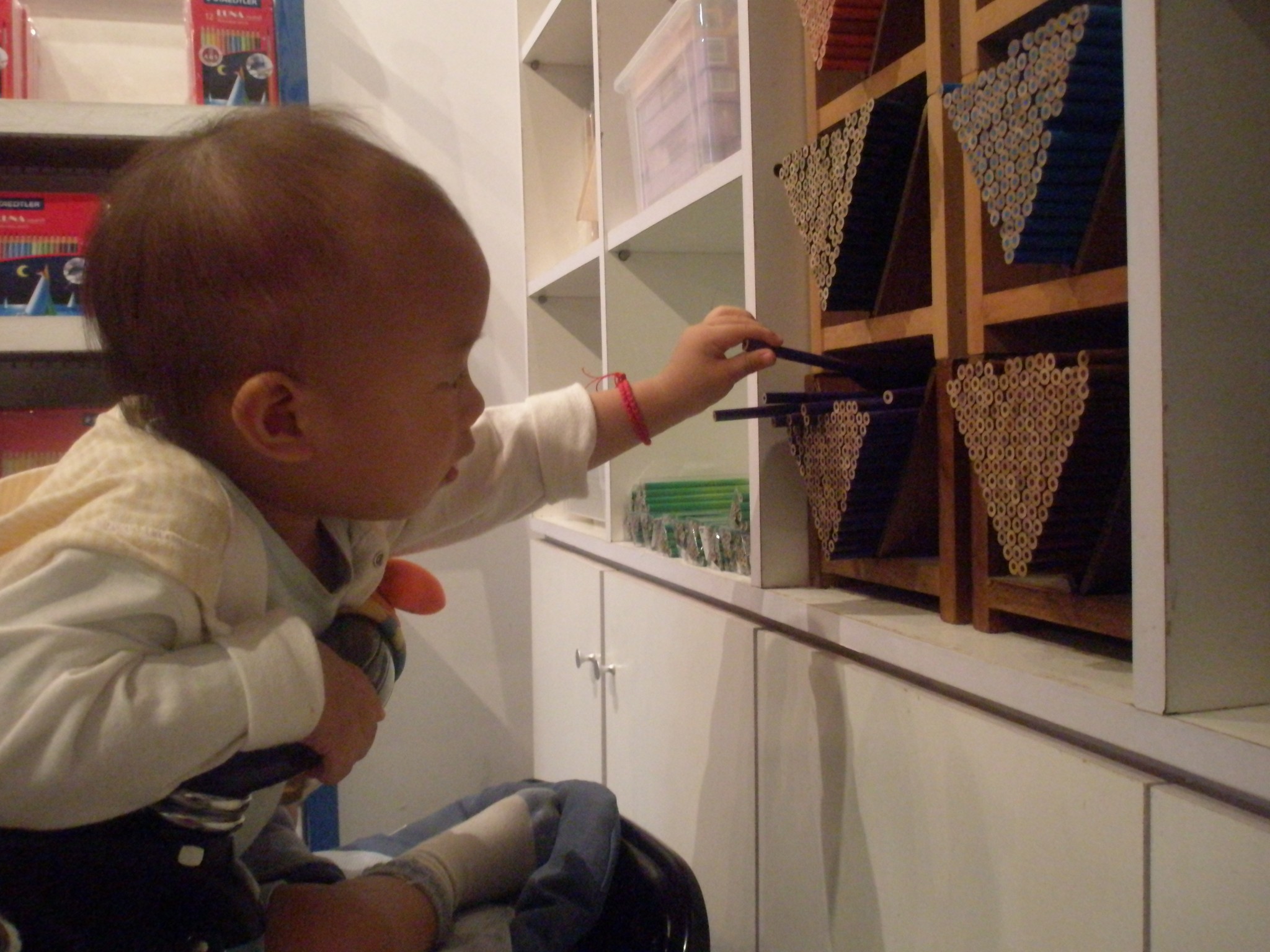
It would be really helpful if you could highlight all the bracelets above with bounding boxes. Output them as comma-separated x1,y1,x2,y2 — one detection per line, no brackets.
581,367,650,445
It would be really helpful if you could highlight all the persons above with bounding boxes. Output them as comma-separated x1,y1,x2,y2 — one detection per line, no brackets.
0,97,784,952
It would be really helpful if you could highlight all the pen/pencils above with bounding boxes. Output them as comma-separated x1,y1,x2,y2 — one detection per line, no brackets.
200,26,261,55
776,97,923,312
742,338,882,376
2,235,79,258
712,384,921,562
945,349,1125,577
936,4,1122,267
795,0,884,73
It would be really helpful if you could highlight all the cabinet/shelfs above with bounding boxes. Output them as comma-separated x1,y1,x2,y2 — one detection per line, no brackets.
1,97,231,350
519,0,1270,952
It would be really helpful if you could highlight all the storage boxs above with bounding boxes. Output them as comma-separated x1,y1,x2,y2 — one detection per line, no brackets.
613,0,738,211
